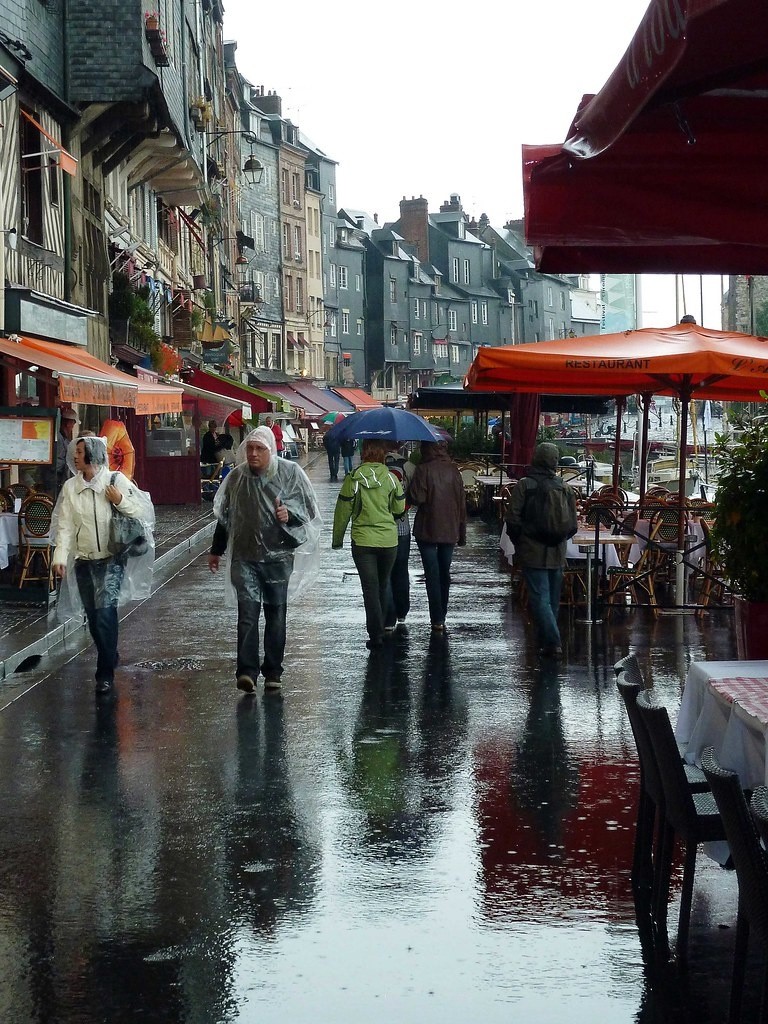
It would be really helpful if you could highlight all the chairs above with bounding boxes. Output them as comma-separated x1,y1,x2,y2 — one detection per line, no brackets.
455,459,507,511
200,457,227,502
0,483,57,592
614,649,768,1024
499,483,729,625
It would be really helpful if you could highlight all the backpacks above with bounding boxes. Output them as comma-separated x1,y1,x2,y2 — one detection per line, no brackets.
525,475,578,546
384,456,411,521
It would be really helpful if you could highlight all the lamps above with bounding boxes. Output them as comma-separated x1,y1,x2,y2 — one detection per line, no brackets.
236,281,266,304
307,309,337,327
211,236,249,264
198,131,264,185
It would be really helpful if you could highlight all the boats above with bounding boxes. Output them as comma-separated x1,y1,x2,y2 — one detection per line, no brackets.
574,453,623,487
628,432,699,498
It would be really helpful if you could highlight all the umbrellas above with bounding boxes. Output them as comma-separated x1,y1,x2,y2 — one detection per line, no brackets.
100,419,135,480
218,434,234,450
407,382,610,484
463,315,768,552
522,0,768,277
509,392,541,482
325,407,445,441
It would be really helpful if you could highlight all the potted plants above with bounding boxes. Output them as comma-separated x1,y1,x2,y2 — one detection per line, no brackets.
707,388,768,659
110,269,166,367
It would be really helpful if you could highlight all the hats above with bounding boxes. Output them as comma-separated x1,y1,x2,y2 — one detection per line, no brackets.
63,408,77,420
208,420,217,427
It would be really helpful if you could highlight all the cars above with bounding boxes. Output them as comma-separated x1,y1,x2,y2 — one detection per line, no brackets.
731,415,768,439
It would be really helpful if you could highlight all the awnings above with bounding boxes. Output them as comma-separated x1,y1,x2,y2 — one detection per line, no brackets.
250,369,383,420
0,335,184,415
205,369,283,413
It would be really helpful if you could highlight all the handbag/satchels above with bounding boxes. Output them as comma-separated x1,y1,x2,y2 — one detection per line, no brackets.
270,518,308,550
108,472,148,556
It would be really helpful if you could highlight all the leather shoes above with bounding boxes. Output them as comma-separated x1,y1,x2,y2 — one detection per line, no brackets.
96,677,112,693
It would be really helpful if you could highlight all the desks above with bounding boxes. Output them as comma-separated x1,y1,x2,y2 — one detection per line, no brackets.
572,533,638,624
0,512,50,584
500,520,626,605
473,475,591,515
616,516,722,578
677,660,768,870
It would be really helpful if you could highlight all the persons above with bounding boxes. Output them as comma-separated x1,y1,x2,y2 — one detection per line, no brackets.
337,632,468,864
48,436,155,691
406,440,467,633
43,408,81,498
332,438,406,651
340,439,355,474
266,416,283,457
201,420,220,480
382,440,416,631
323,435,340,480
228,688,317,938
512,670,580,867
208,426,324,694
505,442,578,669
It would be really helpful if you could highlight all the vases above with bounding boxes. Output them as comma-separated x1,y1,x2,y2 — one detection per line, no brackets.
191,108,206,132
146,17,171,68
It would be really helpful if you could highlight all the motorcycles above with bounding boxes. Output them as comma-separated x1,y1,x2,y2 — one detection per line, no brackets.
594,419,617,438
559,426,587,438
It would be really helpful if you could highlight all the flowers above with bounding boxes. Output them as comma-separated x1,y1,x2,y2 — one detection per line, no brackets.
190,92,211,119
145,9,168,47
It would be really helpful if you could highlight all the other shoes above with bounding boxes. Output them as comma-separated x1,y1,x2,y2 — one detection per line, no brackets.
264,676,281,687
398,617,405,621
237,675,256,693
366,640,383,648
385,626,395,629
